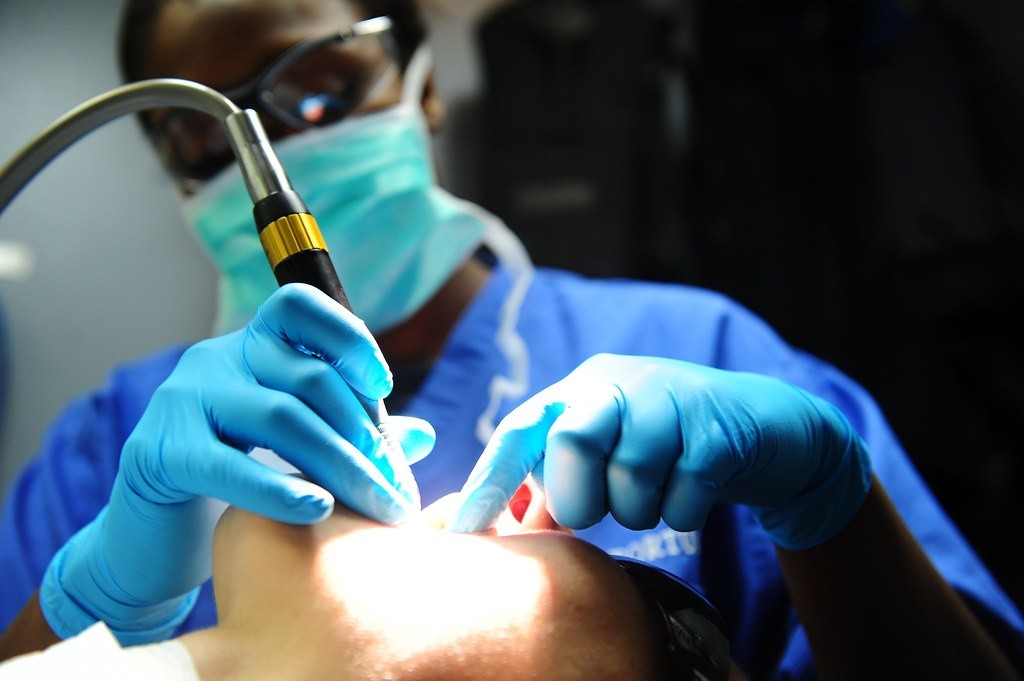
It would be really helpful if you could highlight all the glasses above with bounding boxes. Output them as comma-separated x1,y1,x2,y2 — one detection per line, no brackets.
147,16,396,183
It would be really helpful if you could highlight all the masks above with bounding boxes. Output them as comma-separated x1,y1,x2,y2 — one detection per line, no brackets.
182,44,532,444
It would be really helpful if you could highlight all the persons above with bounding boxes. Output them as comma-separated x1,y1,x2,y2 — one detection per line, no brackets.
1,0,1024,681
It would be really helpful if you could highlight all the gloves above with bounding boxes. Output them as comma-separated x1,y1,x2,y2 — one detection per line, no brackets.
453,352,873,558
38,281,437,650
